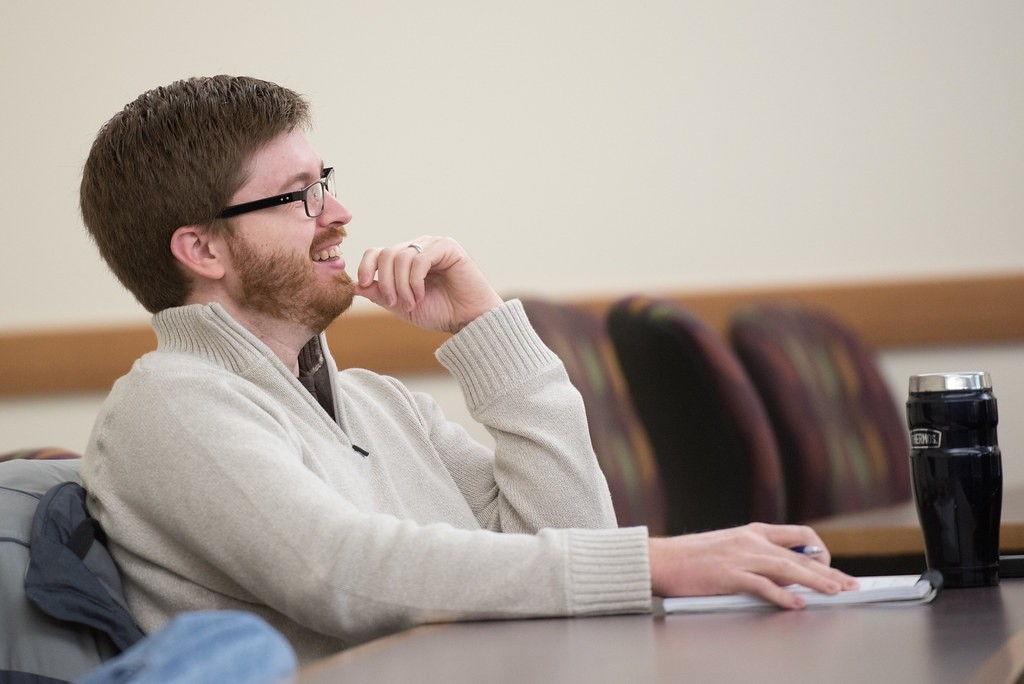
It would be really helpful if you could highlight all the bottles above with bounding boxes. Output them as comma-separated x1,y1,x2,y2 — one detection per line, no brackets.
909,370,1004,586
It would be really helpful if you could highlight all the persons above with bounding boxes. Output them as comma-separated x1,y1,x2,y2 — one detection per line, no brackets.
83,75,860,666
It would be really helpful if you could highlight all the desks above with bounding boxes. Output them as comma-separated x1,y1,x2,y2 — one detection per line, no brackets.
796,487,1023,558
272,575,1024,684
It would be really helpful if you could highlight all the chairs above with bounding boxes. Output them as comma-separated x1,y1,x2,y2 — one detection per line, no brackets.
521,292,915,536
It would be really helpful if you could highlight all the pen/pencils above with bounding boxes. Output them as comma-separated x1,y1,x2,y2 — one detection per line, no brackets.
785,544,825,558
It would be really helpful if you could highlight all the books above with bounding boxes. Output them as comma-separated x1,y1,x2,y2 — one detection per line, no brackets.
661,570,944,614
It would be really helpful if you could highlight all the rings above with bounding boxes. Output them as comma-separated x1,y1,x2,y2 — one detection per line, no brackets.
408,244,423,253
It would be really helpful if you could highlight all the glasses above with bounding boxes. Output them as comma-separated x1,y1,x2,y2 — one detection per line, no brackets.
212,167,336,217
